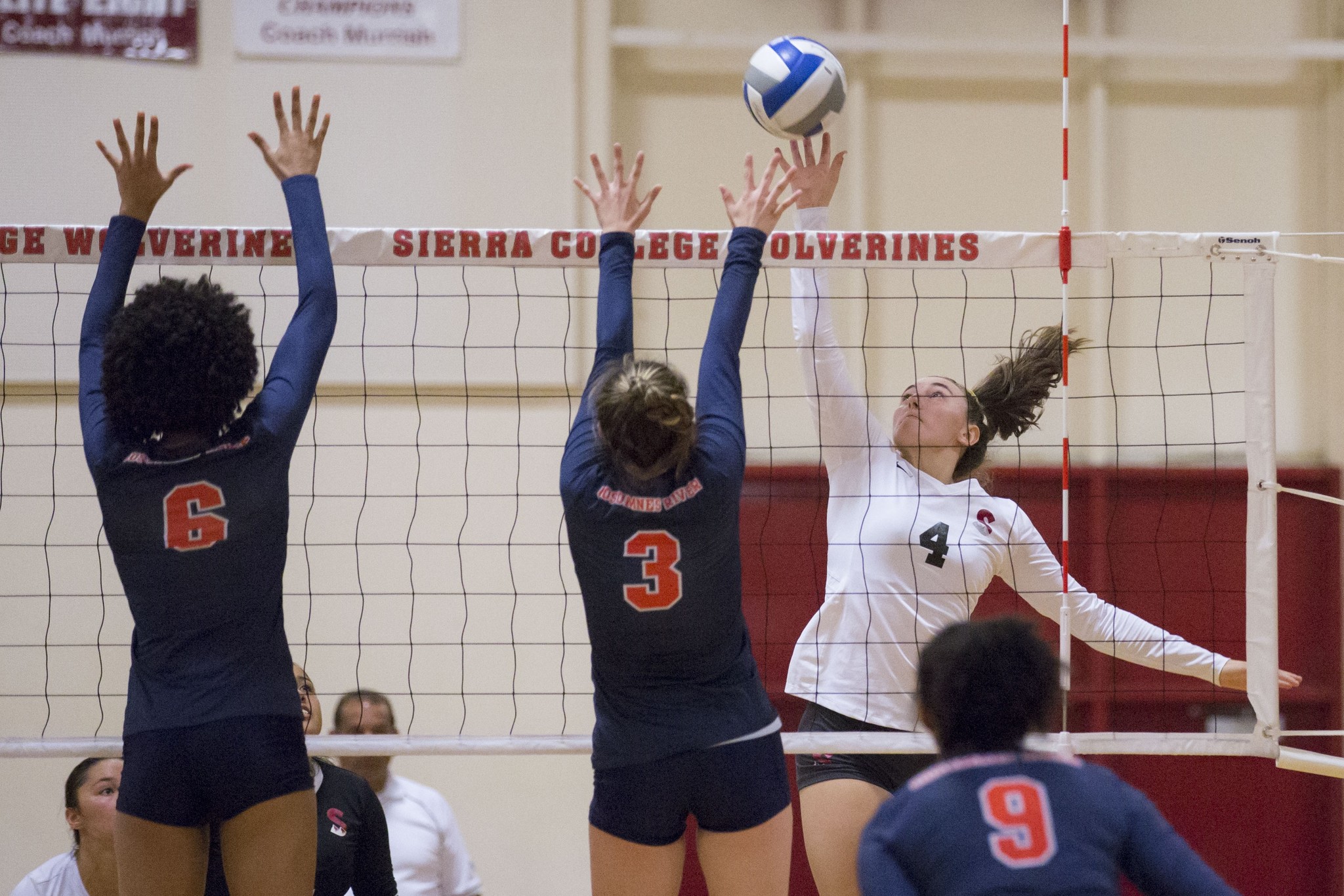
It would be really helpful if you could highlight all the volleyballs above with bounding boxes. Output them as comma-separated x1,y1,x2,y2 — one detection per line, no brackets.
741,35,846,141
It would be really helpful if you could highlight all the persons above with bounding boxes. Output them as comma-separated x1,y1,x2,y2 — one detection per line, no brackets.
78,80,339,896
559,146,801,895
774,130,1300,896
15,662,481,896
